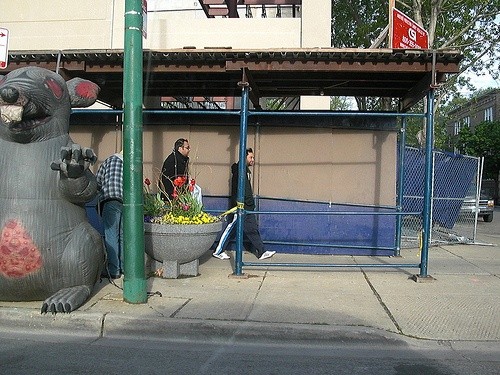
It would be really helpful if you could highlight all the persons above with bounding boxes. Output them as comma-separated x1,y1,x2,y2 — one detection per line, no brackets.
212,148,275,261
158,137,191,206
94,149,125,279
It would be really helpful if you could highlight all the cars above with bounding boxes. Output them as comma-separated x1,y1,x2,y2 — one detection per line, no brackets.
457,178,495,222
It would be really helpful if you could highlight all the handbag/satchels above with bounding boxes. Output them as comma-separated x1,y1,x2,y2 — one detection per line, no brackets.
189,181,202,207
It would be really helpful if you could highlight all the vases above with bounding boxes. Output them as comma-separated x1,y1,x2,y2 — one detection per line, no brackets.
143,220,222,279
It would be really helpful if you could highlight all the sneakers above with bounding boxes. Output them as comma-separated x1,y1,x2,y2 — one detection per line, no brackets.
212,252,230,260
258,251,276,259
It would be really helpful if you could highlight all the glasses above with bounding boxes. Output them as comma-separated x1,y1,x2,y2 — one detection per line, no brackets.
182,146,190,150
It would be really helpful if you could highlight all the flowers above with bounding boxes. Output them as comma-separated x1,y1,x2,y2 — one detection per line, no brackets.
141,149,221,223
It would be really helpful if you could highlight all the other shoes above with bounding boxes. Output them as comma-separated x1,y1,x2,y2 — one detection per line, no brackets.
101,274,120,279
